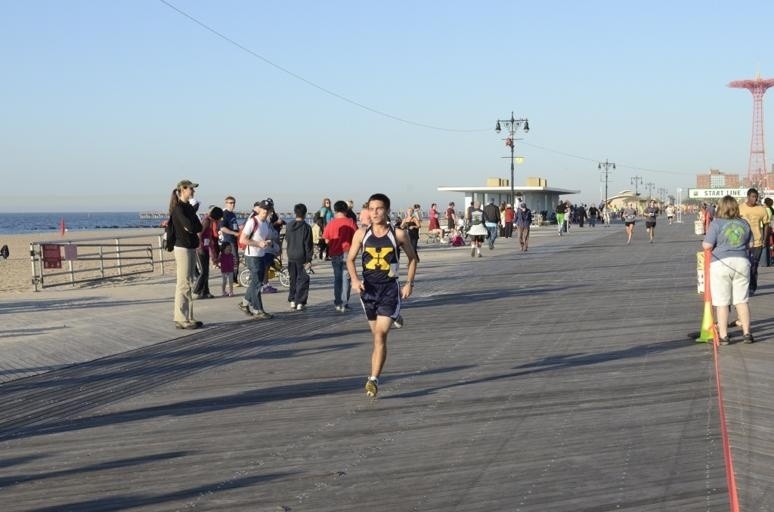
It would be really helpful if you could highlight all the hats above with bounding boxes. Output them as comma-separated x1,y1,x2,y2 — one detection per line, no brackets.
177,180,199,190
259,200,272,210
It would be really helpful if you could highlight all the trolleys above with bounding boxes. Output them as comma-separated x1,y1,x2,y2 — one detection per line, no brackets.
236,233,290,289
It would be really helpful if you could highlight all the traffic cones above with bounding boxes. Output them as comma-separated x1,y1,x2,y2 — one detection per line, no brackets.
694,301,719,344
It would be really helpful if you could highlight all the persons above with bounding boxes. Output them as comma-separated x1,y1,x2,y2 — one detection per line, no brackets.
394,205,421,265
429,201,465,246
698,198,774,266
622,200,674,245
173,181,204,330
738,188,771,297
556,200,621,236
238,197,283,320
285,204,314,311
345,194,417,398
702,196,754,345
313,198,370,312
192,196,240,299
466,197,532,258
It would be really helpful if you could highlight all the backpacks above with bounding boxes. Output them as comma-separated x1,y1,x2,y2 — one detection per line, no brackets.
160,215,176,252
238,217,258,249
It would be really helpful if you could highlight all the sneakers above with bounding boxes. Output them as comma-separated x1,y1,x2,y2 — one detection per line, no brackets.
366,377,378,398
708,337,730,345
391,316,402,329
477,253,482,257
193,321,204,327
236,303,350,319
743,334,753,343
192,286,277,297
176,321,196,331
471,247,475,257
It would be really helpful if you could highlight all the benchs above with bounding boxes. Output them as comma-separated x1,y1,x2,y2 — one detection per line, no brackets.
421,228,469,246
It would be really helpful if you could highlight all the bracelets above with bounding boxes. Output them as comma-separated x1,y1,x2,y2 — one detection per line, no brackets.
407,281,414,287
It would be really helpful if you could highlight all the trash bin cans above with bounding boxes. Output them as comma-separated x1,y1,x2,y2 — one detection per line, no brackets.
694,220,705,235
696,251,705,295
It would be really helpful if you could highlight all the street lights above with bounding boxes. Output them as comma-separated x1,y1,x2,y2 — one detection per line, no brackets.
494,110,530,204
629,173,667,203
597,158,615,210
